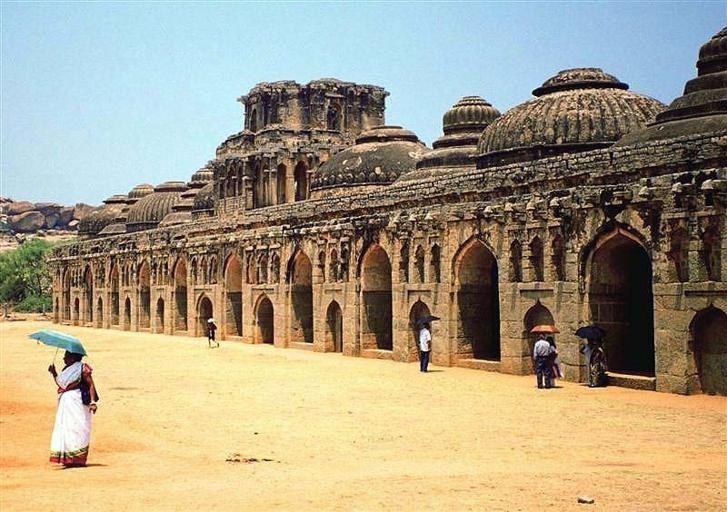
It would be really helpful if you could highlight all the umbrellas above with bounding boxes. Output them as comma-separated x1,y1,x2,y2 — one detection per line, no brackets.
206,318,216,323
530,324,561,334
574,326,606,337
417,315,441,324
28,329,87,375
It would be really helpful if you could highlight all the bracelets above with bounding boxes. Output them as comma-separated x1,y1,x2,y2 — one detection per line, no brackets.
89,401,97,405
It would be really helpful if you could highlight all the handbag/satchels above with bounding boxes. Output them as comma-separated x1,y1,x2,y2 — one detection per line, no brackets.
78,381,98,404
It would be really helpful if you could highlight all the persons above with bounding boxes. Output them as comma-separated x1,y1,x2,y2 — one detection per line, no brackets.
532,333,553,389
546,336,559,387
47,350,100,469
579,337,594,383
590,339,608,387
207,318,219,348
418,322,432,372
